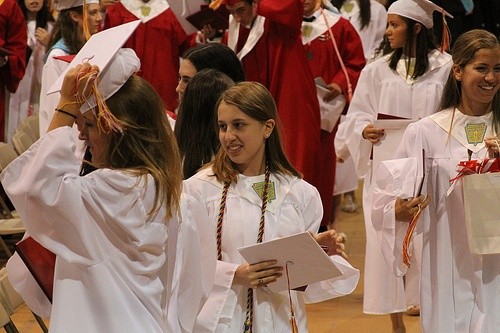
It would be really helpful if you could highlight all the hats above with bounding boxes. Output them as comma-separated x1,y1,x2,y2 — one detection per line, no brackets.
46,20,143,115
387,0,454,29
57,0,100,10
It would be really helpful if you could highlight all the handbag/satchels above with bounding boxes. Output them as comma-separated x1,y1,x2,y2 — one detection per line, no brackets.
462,140,499,255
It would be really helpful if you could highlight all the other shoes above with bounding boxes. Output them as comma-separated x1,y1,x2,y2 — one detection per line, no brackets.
343,196,358,210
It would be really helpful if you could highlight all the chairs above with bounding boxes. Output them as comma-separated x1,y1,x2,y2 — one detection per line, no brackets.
0,113,68,333
